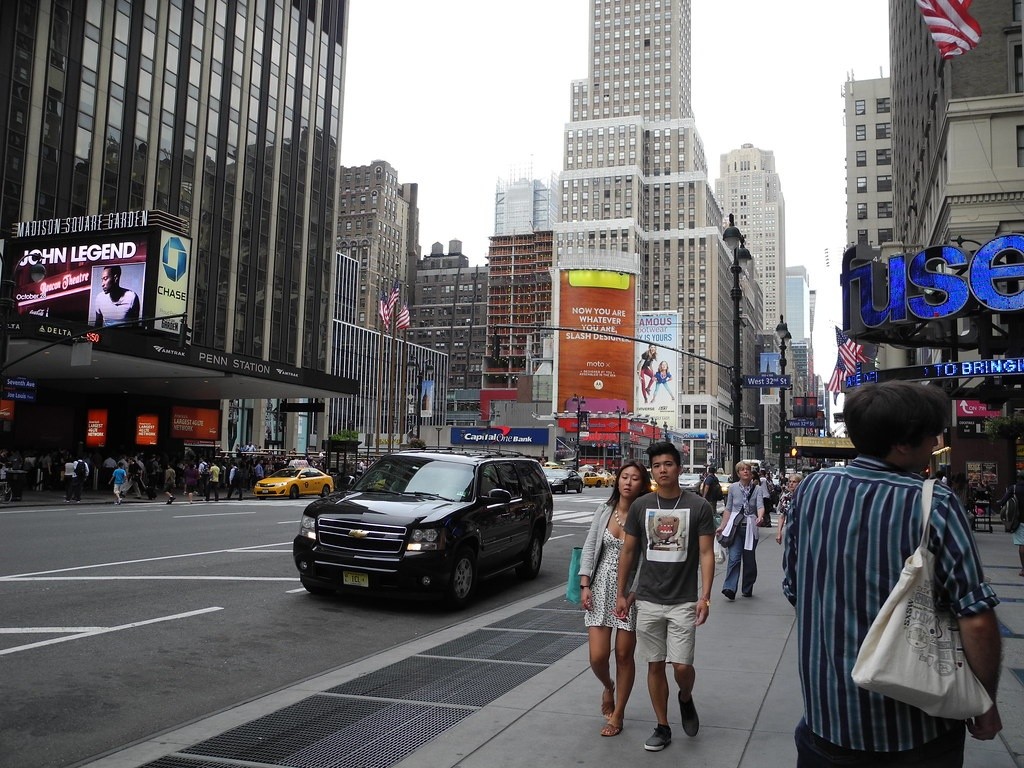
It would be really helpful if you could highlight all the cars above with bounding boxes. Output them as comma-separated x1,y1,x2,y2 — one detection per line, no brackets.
699,472,732,496
544,469,584,495
576,470,607,489
252,468,334,498
679,472,705,497
595,471,614,487
291,449,552,616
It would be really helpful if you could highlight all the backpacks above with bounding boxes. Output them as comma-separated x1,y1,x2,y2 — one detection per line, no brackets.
202,465,210,478
767,480,774,494
75,462,86,475
232,467,242,482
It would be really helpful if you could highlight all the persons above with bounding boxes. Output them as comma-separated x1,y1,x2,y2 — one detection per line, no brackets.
94,265,140,328
918,470,1024,577
540,457,548,466
616,441,716,751
700,461,827,600
636,344,674,403
264,398,273,452
577,461,653,736
228,399,240,452
781,380,1004,768
0,442,374,507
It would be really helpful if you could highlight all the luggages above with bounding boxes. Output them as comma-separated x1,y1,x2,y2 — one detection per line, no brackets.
145,487,157,499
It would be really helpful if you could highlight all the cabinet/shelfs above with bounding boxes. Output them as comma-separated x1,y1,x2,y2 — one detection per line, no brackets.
972,501,993,534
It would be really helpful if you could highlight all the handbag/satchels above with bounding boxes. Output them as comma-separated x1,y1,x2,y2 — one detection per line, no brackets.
130,473,140,481
35,462,43,468
1000,494,1020,533
852,479,993,719
717,514,742,548
703,475,724,501
566,547,582,603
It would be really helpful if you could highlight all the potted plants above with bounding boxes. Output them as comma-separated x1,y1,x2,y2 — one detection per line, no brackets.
983,413,1024,445
322,430,362,454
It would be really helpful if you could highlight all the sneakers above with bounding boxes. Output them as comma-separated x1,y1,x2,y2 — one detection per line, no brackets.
678,691,699,737
645,724,672,751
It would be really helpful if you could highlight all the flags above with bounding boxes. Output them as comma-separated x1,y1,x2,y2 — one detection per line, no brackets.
378,275,410,335
827,326,868,404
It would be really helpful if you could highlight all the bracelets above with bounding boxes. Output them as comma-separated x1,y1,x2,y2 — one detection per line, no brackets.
702,595,711,606
580,585,589,589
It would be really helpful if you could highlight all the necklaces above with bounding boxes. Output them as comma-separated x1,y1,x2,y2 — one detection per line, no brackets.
657,490,683,517
615,505,625,527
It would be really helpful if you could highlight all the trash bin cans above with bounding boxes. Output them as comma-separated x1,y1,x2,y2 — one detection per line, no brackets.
5,471,27,500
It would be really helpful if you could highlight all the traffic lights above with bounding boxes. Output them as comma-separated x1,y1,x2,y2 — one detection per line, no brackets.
179,323,191,353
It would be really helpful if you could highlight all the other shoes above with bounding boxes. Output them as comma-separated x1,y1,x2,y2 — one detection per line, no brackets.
601,724,622,735
722,590,735,600
63,500,69,503
602,682,615,719
167,496,175,504
72,497,80,503
201,496,243,502
742,592,751,597
114,498,122,505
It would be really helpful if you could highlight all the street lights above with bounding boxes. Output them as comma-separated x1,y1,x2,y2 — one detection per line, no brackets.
663,422,668,442
650,418,658,443
722,210,752,526
571,393,586,470
775,313,791,487
405,352,434,452
614,407,628,473
